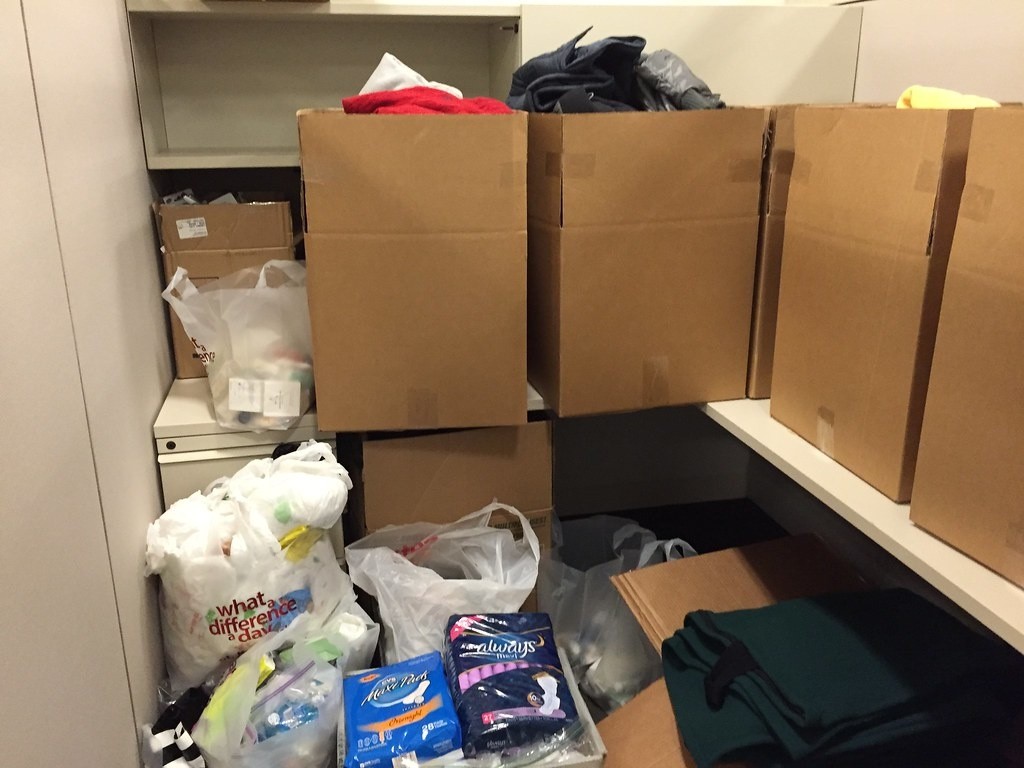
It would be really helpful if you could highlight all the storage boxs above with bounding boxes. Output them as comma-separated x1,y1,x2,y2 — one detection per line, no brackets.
747,102,887,400
591,532,848,768
531,107,766,418
770,106,974,503
296,105,529,433
909,107,1024,592
348,420,553,615
148,201,300,380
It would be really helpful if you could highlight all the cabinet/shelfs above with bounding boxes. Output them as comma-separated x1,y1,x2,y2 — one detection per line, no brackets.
117,0,1022,650
